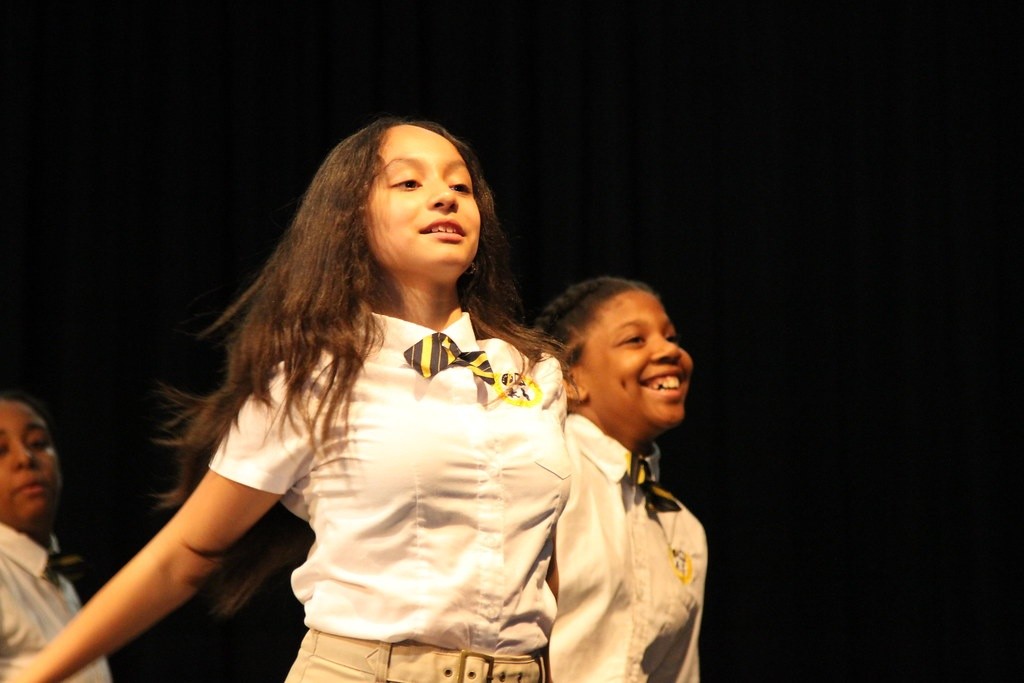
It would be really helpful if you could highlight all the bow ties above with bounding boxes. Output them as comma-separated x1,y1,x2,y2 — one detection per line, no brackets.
403,332,495,386
624,451,681,514
41,554,82,588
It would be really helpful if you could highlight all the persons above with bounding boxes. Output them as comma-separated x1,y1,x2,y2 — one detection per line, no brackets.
0,111,574,682
529,277,707,683
0,389,111,682
